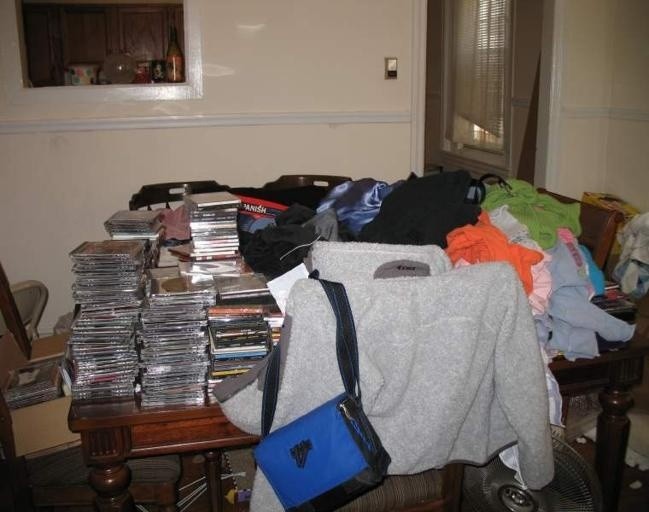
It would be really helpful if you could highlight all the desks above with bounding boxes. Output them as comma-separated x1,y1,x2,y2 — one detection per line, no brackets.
69,263,643,508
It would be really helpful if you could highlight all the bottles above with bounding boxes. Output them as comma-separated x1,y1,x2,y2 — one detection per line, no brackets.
165,26,184,84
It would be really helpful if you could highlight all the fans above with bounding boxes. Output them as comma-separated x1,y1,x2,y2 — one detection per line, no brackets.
458,418,606,509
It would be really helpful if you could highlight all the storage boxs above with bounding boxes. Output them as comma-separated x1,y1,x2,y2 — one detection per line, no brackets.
0,330,93,486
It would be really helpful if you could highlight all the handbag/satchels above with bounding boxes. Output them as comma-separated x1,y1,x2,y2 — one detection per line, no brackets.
251,269,391,512
